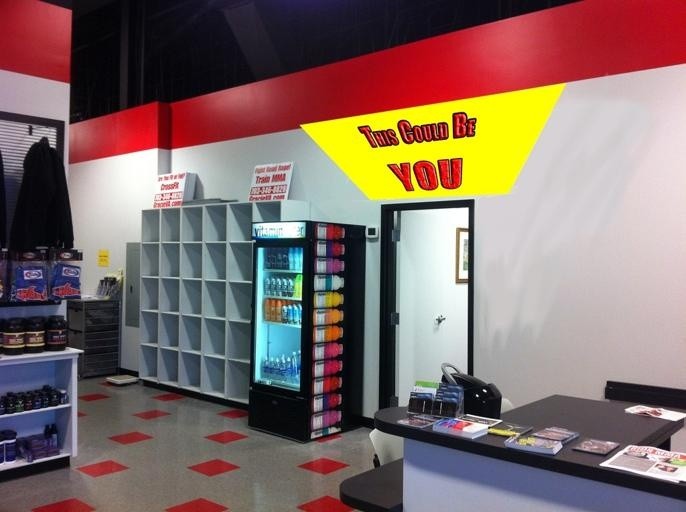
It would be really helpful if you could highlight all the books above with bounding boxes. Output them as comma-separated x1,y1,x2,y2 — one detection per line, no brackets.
624,404,686,422
572,438,620,455
598,445,686,484
457,414,502,427
531,426,579,443
504,436,563,455
489,422,534,436
433,417,489,439
397,415,442,428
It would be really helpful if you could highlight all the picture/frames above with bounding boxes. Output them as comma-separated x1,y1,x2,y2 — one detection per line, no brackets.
456,227,468,283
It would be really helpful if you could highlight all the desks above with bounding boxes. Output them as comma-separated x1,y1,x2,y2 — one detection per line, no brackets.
339,394,686,511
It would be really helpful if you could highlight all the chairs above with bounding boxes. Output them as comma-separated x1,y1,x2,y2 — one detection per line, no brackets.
604,379,685,453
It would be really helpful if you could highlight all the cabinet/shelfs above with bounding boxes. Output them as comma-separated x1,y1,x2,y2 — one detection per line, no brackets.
66,296,121,380
140,199,311,410
1,248,85,484
373,404,686,512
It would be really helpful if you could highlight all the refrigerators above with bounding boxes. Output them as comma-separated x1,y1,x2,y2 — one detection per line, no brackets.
246,221,367,444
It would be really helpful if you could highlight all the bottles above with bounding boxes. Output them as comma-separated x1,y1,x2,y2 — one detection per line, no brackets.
288,300,293,304
289,247,294,270
314,274,344,291
1,385,55,404
313,308,343,326
276,277,281,296
25,400,33,410
44,424,49,448
311,361,342,378
8,404,15,413
0,405,5,415
312,344,343,361
45,316,69,351
262,353,292,382
59,389,69,404
51,423,58,452
265,276,270,296
265,251,288,269
42,398,50,408
295,247,299,269
51,393,59,406
288,278,296,296
311,394,343,413
276,301,281,322
0,429,16,441
265,299,271,321
313,326,343,343
4,439,16,460
281,277,286,296
16,401,24,413
271,300,277,322
311,427,343,439
0,319,5,354
26,317,45,353
0,442,4,463
300,247,304,272
57,389,62,402
32,398,42,409
310,410,342,430
294,275,303,299
2,318,25,354
316,241,345,256
317,225,344,239
271,276,276,297
314,292,344,307
257,223,305,238
312,377,343,395
315,258,344,274
292,350,300,383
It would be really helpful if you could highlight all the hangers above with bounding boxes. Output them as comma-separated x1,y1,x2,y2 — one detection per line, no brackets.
31,126,56,151
